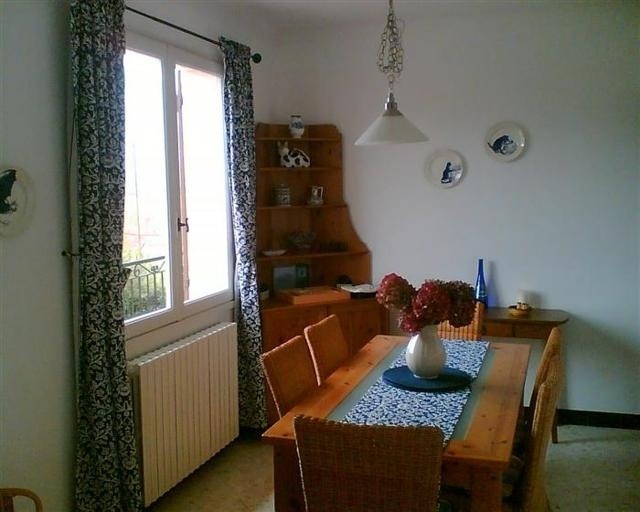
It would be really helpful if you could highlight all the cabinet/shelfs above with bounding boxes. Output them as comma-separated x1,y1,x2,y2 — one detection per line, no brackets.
257,124,373,290
259,300,389,424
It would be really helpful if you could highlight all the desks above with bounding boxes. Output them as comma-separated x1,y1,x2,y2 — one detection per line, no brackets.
482,306,573,443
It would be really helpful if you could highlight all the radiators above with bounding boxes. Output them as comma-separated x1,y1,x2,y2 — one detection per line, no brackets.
123,317,239,504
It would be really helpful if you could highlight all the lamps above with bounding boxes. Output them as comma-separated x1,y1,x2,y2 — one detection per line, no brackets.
351,1,431,147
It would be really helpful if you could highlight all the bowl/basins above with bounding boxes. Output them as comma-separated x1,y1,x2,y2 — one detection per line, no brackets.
508,306,531,317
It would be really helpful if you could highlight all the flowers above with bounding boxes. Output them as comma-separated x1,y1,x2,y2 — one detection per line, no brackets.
374,272,478,335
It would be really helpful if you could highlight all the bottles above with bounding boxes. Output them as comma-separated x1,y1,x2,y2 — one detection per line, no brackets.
516,301,528,310
289,113,305,138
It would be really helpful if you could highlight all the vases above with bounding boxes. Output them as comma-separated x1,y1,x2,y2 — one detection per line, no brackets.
404,318,449,381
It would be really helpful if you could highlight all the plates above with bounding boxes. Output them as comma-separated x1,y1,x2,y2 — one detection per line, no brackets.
262,249,287,257
0,166,38,239
486,122,526,161
337,281,380,294
426,149,463,188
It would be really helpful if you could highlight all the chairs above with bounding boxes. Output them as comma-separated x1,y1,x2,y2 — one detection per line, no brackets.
511,354,564,511
513,325,564,450
300,314,351,384
261,331,322,416
0,488,46,511
290,413,445,510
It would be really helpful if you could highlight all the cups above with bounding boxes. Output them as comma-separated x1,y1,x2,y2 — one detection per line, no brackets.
306,185,324,205
259,290,270,303
273,183,291,206
